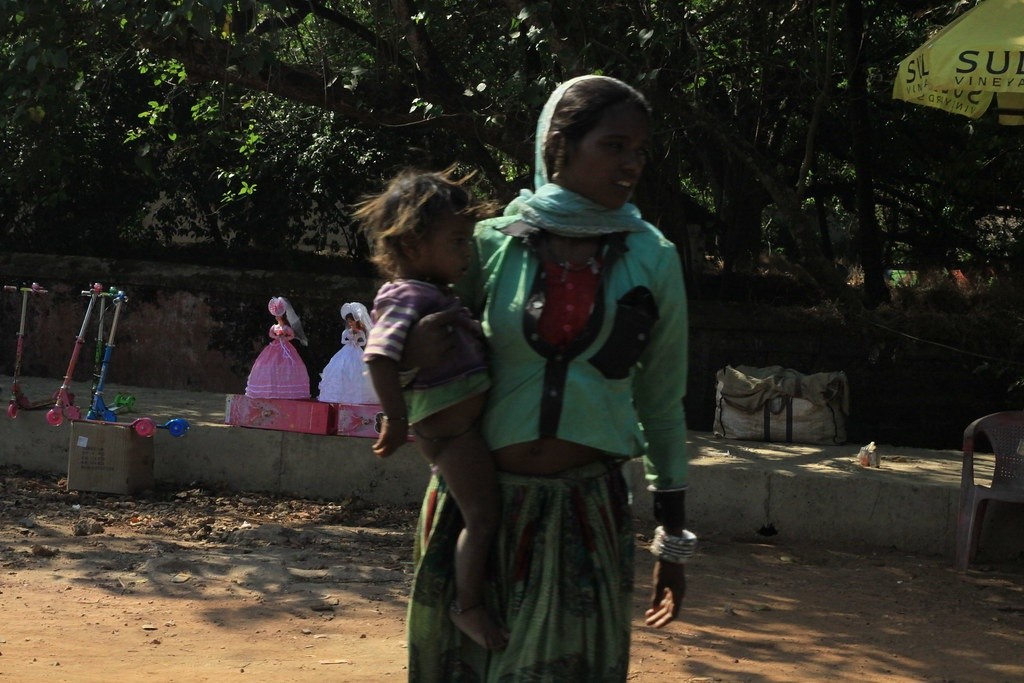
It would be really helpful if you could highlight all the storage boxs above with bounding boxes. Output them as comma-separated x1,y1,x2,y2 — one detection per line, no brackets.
330,403,415,438
222,395,329,433
67,421,155,496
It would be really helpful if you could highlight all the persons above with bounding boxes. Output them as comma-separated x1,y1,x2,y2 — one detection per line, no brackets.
362,169,512,655
320,301,375,404
265,298,298,399
368,75,703,683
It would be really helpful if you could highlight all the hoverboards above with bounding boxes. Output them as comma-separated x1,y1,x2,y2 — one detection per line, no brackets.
45,282,156,438
82,288,138,419
85,295,189,437
4,282,76,417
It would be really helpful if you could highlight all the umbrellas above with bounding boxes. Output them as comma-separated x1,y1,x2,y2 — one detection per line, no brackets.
890,1,1024,126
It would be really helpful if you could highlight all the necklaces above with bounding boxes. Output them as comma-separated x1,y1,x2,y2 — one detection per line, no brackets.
547,241,609,274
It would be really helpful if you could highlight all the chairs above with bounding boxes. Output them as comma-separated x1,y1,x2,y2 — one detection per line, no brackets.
954,410,1024,571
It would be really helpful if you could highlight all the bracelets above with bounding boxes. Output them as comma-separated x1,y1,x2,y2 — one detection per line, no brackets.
648,527,702,565
383,416,407,421
